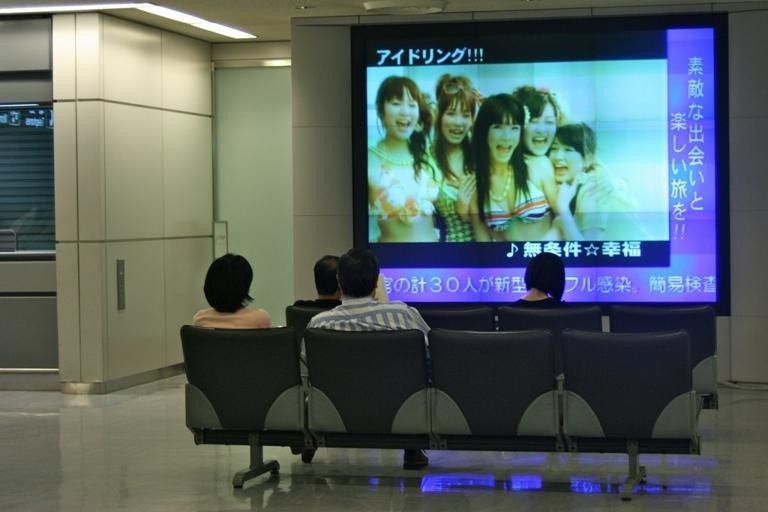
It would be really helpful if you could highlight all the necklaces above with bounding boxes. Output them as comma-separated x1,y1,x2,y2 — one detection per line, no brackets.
369,143,420,166
487,166,512,202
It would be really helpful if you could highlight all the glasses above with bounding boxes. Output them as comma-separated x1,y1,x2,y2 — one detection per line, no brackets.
437,82,481,102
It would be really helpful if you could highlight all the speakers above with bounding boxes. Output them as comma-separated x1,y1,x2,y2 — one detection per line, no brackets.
212,220,230,259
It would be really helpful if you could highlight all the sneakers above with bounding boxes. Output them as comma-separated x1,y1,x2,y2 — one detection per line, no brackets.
302,450,315,463
403,449,429,470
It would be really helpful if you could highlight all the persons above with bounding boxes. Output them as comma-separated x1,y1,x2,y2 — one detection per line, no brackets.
185,254,316,465
546,121,611,240
421,74,498,250
367,74,446,245
500,254,577,313
296,253,344,311
467,94,587,242
299,250,431,474
456,83,612,246
